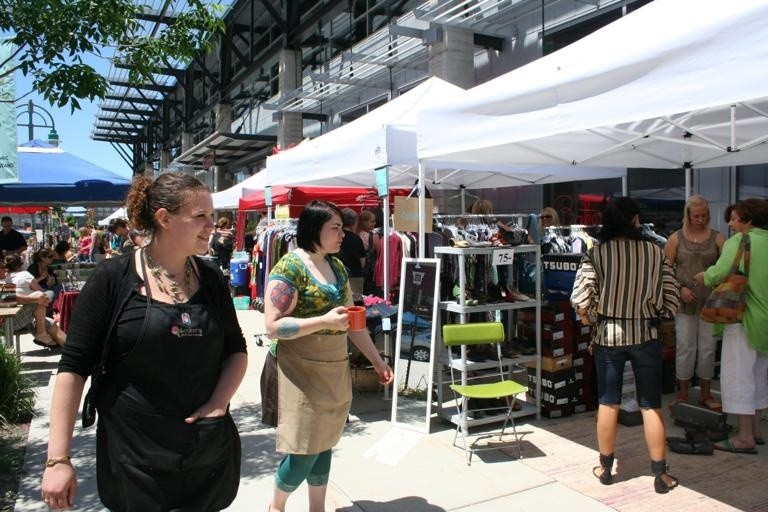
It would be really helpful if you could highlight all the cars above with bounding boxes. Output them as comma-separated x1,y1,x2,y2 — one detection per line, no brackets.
401,332,432,361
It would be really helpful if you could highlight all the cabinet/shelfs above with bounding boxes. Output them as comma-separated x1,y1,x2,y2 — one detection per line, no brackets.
433,245,542,437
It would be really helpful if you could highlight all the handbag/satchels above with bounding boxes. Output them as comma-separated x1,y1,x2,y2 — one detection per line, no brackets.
699,230,751,324
0,283,18,308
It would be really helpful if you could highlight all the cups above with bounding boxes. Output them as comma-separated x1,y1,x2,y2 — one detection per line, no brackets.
343,305,367,333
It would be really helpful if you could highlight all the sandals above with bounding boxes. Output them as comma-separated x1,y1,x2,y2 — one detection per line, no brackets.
591,452,616,486
34,334,59,348
446,230,510,248
699,392,722,409
649,459,680,494
668,394,688,409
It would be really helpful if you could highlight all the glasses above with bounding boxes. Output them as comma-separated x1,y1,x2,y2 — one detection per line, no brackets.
18,262,24,267
45,254,55,259
540,214,555,219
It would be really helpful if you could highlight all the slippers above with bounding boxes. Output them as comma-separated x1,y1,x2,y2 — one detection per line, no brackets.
710,438,758,452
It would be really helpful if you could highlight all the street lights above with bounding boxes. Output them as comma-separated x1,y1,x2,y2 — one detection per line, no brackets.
16,100,60,149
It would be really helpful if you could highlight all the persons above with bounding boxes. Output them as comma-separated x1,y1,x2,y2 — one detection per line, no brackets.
0,216,27,260
105,216,146,256
91,224,107,263
332,207,366,295
74,227,92,263
664,195,727,412
40,173,249,512
27,248,62,299
540,206,561,228
472,199,493,214
52,241,71,263
690,198,768,454
4,255,54,302
87,223,95,233
208,215,237,285
359,211,381,294
569,195,680,494
265,199,393,512
0,263,67,348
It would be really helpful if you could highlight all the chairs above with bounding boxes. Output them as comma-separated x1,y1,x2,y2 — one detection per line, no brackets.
442,321,531,464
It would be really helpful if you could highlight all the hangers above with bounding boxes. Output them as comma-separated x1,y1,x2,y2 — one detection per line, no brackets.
543,224,603,244
431,212,542,242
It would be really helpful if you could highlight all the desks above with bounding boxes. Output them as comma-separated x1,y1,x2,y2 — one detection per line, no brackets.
0,304,24,347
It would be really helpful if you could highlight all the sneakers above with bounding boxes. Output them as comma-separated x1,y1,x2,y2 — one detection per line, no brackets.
511,336,536,355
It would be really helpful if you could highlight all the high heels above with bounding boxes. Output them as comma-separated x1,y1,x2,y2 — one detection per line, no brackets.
452,284,478,306
496,280,518,302
507,280,530,302
